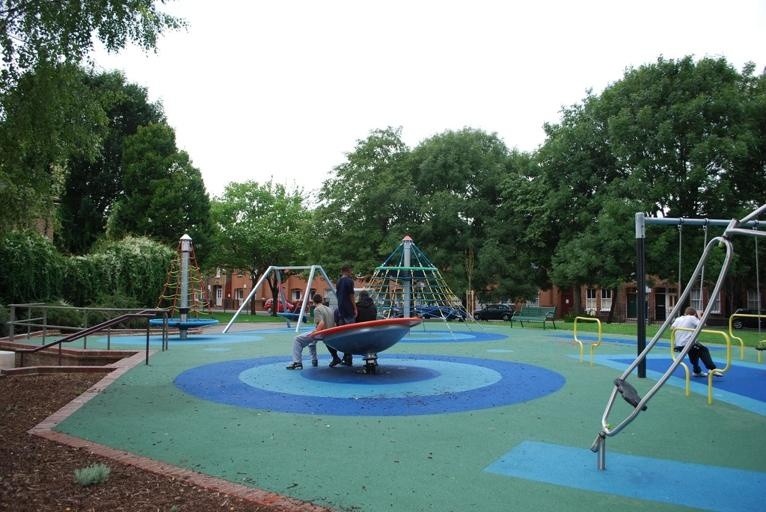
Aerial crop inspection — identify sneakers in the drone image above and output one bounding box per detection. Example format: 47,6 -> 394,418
328,357 -> 341,367
708,369 -> 722,377
312,360 -> 318,368
286,362 -> 302,370
692,371 -> 708,377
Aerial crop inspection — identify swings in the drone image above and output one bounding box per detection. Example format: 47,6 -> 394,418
753,220 -> 766,350
672,218 -> 709,352
273,266 -> 308,319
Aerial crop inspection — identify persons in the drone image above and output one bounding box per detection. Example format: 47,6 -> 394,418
286,265 -> 378,370
670,307 -> 726,378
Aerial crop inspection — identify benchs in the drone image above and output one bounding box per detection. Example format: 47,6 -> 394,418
510,306 -> 557,330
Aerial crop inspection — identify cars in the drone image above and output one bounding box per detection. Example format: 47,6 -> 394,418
264,298 -> 295,313
474,303 -> 514,321
732,308 -> 766,329
